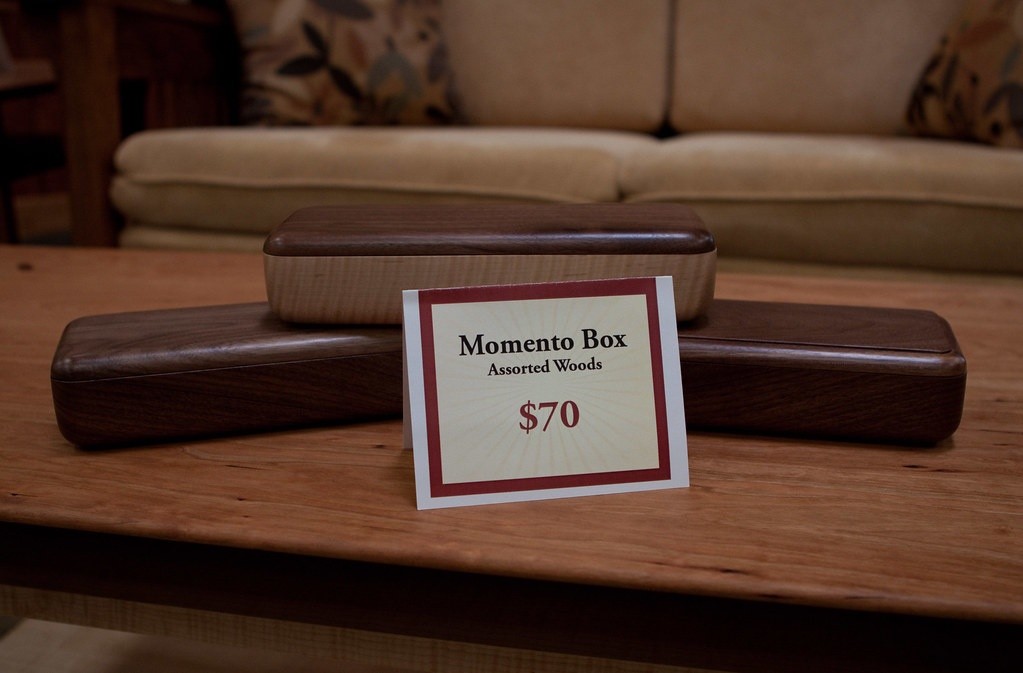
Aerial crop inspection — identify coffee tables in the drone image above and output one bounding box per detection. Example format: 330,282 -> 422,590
1,247 -> 1023,673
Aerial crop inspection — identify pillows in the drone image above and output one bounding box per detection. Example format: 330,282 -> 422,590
896,0 -> 1023,150
230,0 -> 466,127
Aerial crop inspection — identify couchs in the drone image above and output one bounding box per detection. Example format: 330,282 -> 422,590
108,0 -> 1023,274
